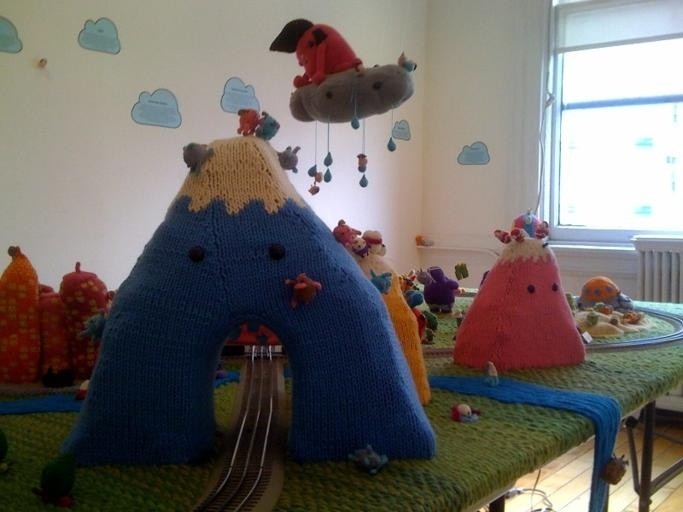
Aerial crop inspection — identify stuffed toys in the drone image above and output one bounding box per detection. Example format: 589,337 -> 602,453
1,19 -> 649,461
1,18 -> 648,462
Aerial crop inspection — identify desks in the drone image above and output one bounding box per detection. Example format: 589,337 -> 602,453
1,279 -> 682,512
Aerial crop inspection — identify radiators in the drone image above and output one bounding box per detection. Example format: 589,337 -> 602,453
631,232 -> 683,399
